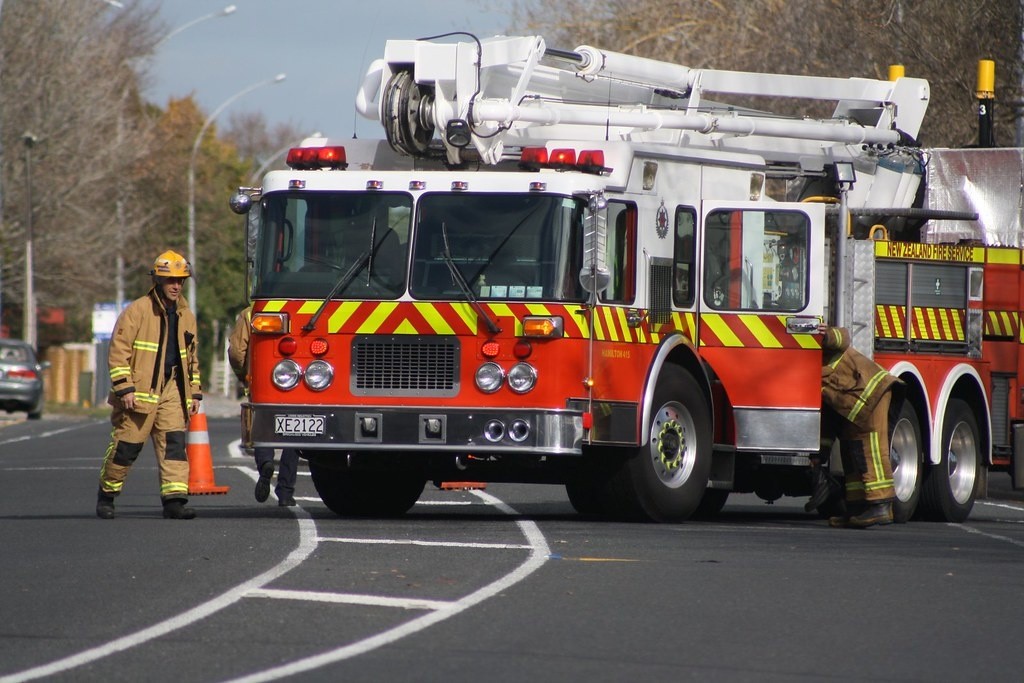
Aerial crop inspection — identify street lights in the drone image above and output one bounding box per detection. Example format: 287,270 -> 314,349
110,5 -> 242,323
181,70 -> 324,321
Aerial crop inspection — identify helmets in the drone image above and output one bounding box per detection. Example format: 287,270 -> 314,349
151,250 -> 191,278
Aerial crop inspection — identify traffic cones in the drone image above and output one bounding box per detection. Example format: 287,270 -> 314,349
188,399 -> 225,495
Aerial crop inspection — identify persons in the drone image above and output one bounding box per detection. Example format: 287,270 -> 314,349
226,275 -> 300,507
96,250 -> 202,518
809,321 -> 898,528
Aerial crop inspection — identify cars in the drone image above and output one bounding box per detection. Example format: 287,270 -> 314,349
0,339 -> 53,418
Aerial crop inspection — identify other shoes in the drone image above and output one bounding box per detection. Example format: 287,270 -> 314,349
255,462 -> 274,502
849,501 -> 894,527
96,489 -> 115,519
163,499 -> 196,519
279,497 -> 296,506
829,503 -> 864,528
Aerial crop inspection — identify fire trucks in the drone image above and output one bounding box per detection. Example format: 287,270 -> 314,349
228,28 -> 1024,529
